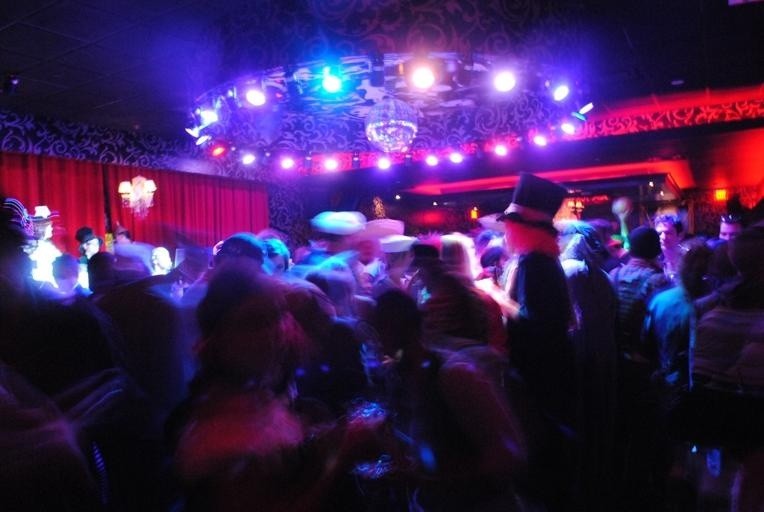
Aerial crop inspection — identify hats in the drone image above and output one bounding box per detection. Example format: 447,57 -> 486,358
496,171 -> 568,238
76,227 -> 103,248
355,219 -> 405,241
379,235 -> 418,252
28,204 -> 61,221
0,196 -> 46,239
310,210 -> 367,235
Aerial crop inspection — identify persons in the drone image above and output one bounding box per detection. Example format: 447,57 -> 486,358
1,170 -> 763,511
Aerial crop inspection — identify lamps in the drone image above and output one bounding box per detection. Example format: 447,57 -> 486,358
117,157 -> 157,221
239,148 -> 255,165
184,120 -> 206,137
363,92 -> 419,153
207,139 -> 226,159
543,77 -> 569,101
574,95 -> 594,115
192,132 -> 209,145
